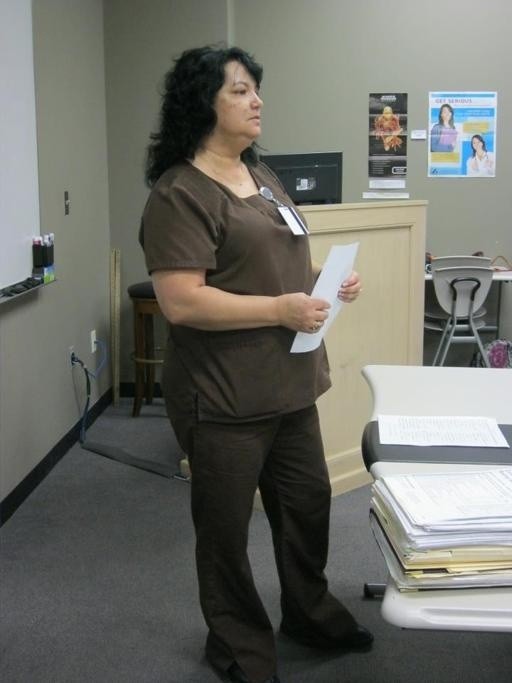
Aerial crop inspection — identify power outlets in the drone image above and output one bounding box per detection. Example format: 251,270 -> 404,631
91,330 -> 98,352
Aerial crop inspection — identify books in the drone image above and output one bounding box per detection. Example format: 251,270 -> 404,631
368,466 -> 512,594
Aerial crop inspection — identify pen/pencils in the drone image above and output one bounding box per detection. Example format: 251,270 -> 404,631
32,232 -> 55,279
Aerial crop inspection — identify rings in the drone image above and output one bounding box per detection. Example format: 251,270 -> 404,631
313,322 -> 320,332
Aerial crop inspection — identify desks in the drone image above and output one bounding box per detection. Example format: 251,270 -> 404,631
180,199 -> 433,510
426,255 -> 512,368
359,364 -> 512,642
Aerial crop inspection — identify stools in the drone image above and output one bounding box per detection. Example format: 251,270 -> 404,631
127,280 -> 168,418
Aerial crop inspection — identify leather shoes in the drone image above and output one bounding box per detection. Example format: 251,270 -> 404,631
205,653 -> 278,682
280,614 -> 373,653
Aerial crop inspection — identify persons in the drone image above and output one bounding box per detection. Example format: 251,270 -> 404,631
137,42 -> 375,682
431,104 -> 494,176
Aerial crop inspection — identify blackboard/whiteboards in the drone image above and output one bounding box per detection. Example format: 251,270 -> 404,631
0,0 -> 54,303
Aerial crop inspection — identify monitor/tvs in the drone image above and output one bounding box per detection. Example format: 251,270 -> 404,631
259,152 -> 343,206
